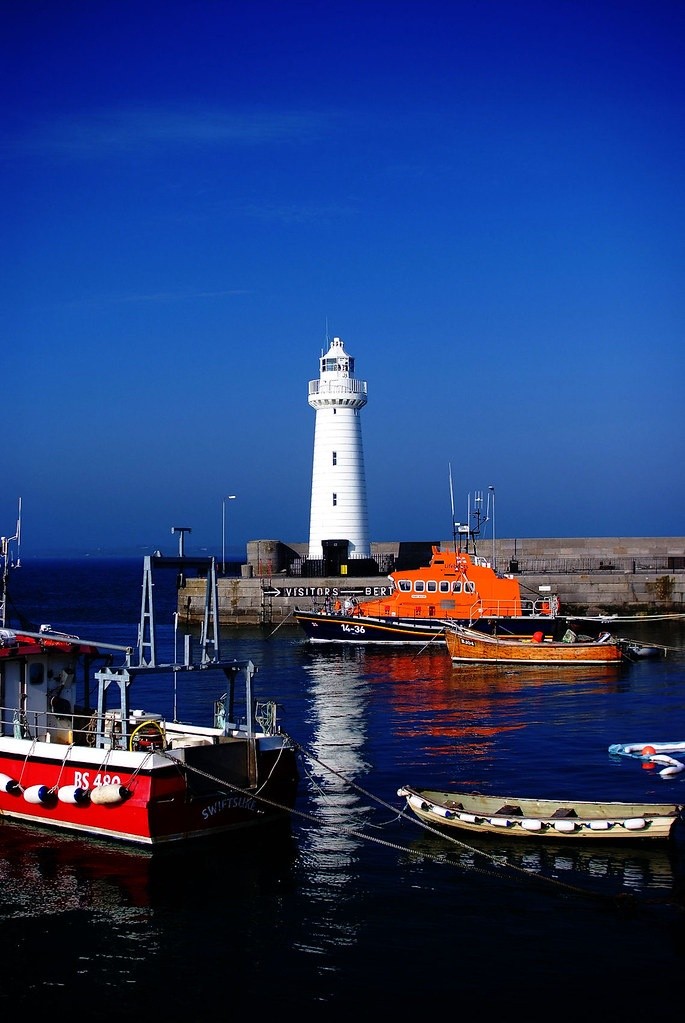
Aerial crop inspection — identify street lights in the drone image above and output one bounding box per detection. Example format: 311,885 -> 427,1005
488,485 -> 497,571
222,496 -> 237,574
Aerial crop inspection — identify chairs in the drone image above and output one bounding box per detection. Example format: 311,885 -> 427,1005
596,632 -> 611,642
531,631 -> 544,643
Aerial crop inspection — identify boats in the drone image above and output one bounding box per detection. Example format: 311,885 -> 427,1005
439,618 -> 661,666
0,498 -> 300,851
396,784 -> 685,842
291,462 -> 685,652
609,740 -> 685,776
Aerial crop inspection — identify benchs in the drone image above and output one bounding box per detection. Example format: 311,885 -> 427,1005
494,804 -> 519,815
444,800 -> 460,808
550,808 -> 574,817
